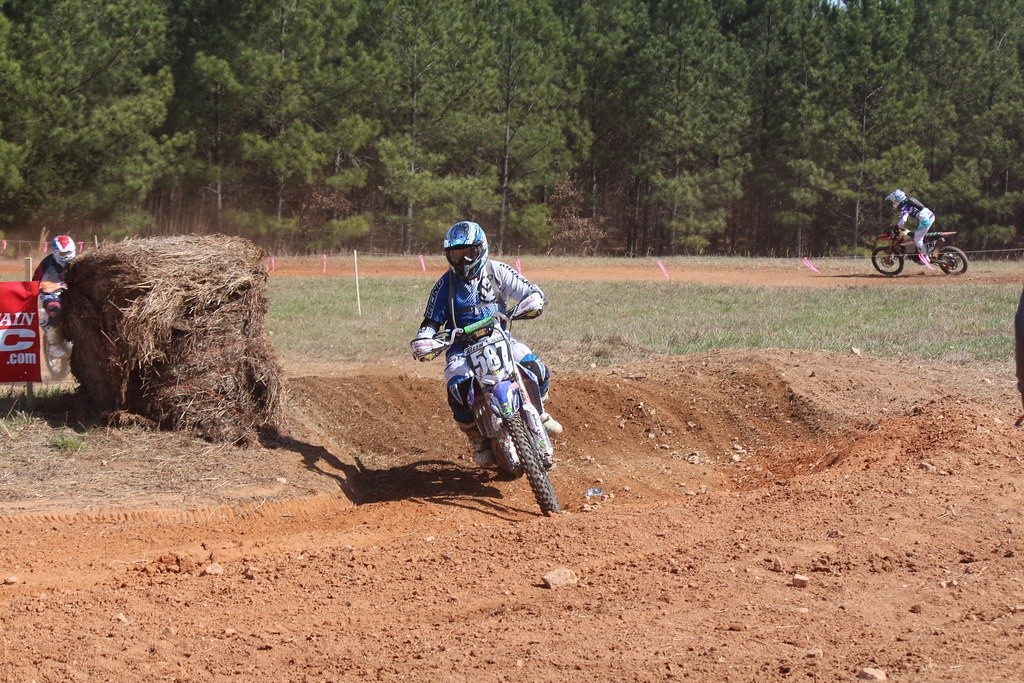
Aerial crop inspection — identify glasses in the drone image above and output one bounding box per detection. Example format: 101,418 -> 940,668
445,242 -> 483,267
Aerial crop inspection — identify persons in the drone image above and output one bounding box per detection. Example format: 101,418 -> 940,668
412,221 -> 562,469
1014,287 -> 1024,425
32,235 -> 76,329
886,189 -> 935,264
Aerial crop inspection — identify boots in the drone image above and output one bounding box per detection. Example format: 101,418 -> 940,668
538,387 -> 563,435
456,420 -> 495,469
916,245 -> 930,264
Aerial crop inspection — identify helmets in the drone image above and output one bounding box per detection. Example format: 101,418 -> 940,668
884,188 -> 909,211
52,234 -> 75,269
443,220 -> 489,281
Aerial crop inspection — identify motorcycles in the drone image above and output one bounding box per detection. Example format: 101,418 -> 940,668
870,215 -> 969,275
413,298 -> 561,517
40,282 -> 72,380
